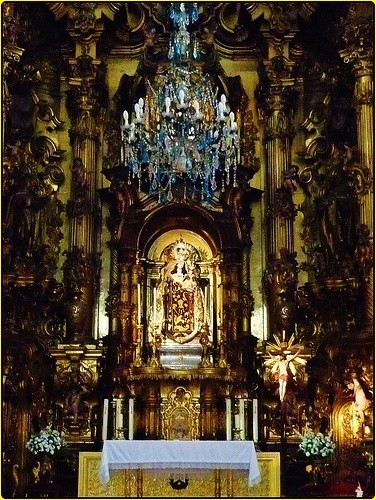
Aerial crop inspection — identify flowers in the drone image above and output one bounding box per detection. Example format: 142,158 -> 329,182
296,429 -> 335,457
25,429 -> 63,454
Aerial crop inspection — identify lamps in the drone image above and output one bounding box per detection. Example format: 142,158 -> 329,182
118,2 -> 241,204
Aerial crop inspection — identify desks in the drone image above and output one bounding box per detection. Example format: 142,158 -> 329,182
100,440 -> 261,497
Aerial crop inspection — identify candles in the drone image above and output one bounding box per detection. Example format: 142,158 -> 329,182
239,399 -> 244,440
252,399 -> 258,443
103,399 -> 109,441
226,399 -> 232,440
116,400 -> 123,428
128,399 -> 134,440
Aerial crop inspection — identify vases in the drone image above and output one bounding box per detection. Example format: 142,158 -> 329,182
29,452 -> 63,498
301,459 -> 332,498
